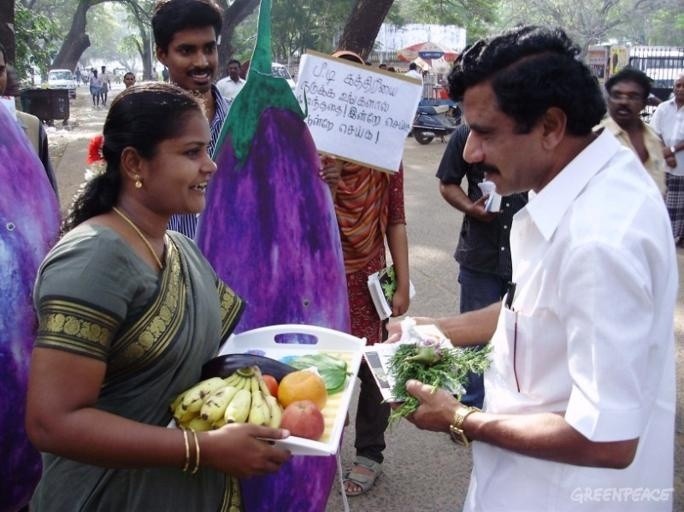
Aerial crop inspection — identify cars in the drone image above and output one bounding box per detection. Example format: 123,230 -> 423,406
81,65 -> 144,81
270,62 -> 296,99
17,66 -> 41,89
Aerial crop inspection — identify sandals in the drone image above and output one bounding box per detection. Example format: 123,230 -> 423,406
340,456 -> 382,496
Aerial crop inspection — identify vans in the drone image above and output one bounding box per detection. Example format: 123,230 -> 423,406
45,69 -> 76,100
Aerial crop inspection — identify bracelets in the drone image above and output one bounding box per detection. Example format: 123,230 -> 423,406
187,427 -> 200,475
182,430 -> 190,473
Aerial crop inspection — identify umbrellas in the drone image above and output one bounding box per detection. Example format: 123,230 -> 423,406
396,41 -> 459,73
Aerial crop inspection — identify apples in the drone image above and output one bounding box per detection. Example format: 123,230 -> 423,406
278,400 -> 325,440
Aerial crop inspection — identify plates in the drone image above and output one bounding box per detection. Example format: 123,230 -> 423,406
163,323 -> 367,459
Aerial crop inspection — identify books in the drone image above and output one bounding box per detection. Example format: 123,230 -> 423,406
367,265 -> 416,321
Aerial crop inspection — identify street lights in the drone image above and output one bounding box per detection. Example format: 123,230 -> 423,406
147,27 -> 154,82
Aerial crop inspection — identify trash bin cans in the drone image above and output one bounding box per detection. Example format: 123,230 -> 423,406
21,88 -> 70,126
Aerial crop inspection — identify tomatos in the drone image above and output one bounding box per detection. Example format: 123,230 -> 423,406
263,375 -> 278,397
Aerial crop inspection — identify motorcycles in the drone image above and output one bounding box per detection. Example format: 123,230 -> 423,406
410,104 -> 465,145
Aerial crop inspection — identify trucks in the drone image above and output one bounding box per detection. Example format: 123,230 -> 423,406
629,45 -> 683,116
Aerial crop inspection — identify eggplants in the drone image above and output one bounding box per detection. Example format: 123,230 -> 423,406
202,353 -> 299,382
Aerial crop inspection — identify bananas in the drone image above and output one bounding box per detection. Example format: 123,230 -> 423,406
168,366 -> 282,431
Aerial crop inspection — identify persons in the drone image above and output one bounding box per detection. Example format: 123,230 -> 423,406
0,45 -> 60,206
26,80 -> 293,512
152,0 -> 340,241
436,125 -> 529,408
598,68 -> 667,198
75,60 -> 449,137
649,76 -> 684,247
383,27 -> 679,512
291,50 -> 410,497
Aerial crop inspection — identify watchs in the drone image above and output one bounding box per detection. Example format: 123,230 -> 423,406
449,407 -> 481,447
671,144 -> 675,154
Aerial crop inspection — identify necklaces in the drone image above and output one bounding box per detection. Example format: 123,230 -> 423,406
112,207 -> 163,270
